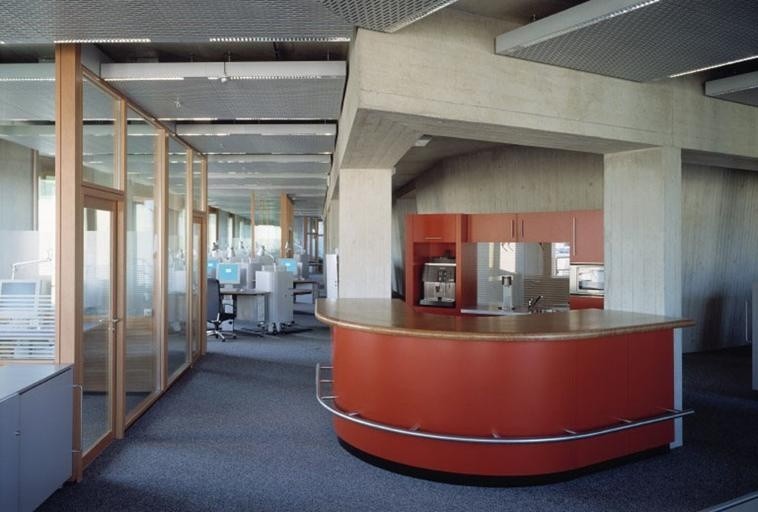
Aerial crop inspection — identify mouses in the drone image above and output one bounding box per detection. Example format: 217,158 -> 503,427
28,326 -> 40,330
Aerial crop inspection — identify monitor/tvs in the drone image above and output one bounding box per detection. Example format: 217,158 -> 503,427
0,279 -> 41,328
276,257 -> 298,276
216,262 -> 240,292
204,259 -> 220,273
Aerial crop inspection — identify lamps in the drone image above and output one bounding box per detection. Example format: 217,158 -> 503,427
210,240 -> 304,261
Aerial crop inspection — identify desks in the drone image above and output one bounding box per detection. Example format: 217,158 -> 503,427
223,278 -> 318,335
1,322 -> 99,355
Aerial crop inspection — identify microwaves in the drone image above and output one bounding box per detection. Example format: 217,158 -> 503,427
568,265 -> 605,296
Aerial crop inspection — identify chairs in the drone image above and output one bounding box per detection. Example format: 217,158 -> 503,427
207,278 -> 235,342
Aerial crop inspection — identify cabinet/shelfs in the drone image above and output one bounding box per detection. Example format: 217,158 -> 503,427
571,210 -> 604,265
413,215 -> 458,312
467,212 -> 570,242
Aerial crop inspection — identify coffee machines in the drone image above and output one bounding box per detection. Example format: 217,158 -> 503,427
419,249 -> 456,307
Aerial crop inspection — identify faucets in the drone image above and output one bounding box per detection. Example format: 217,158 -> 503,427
527,295 -> 544,314
